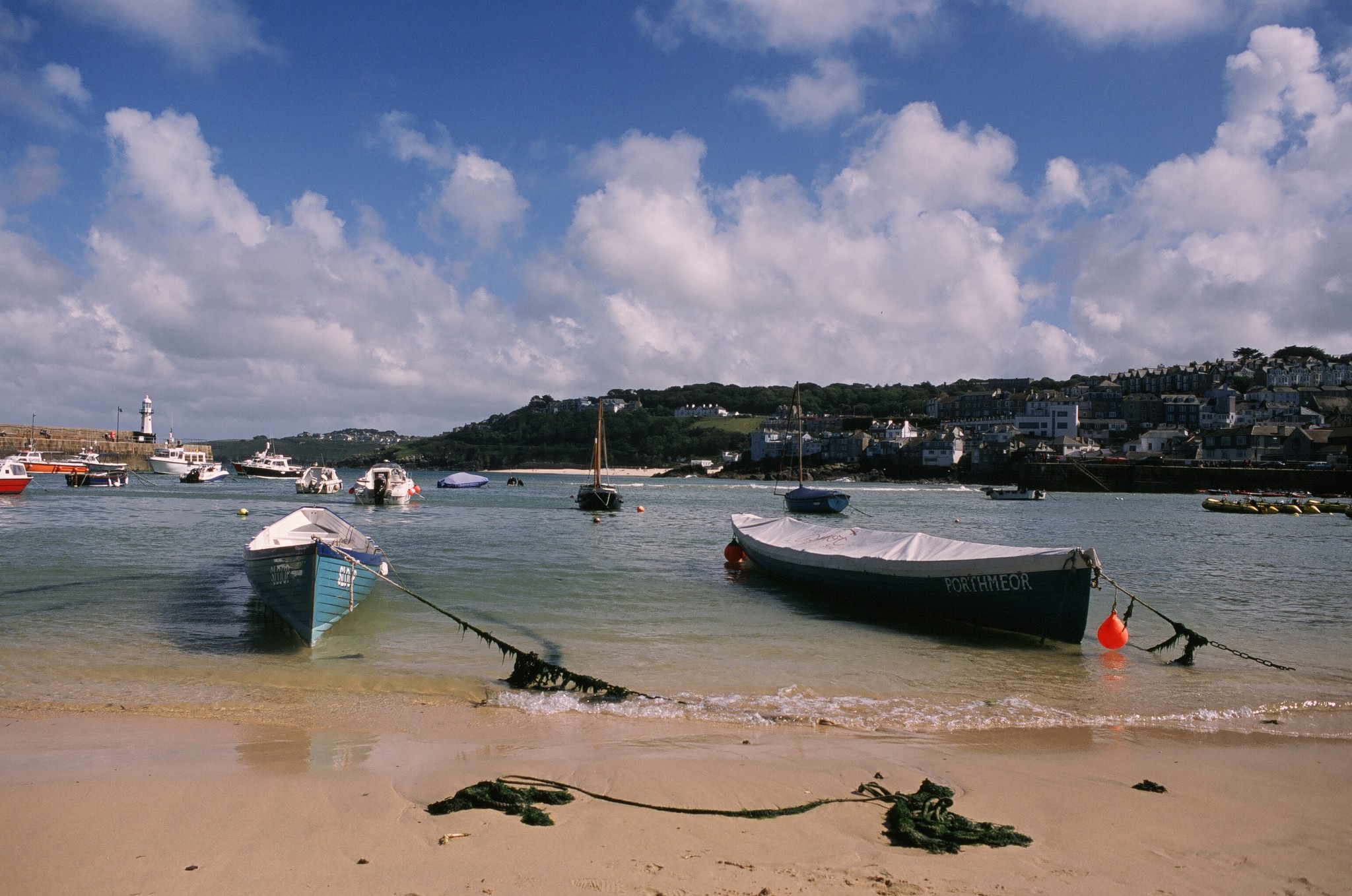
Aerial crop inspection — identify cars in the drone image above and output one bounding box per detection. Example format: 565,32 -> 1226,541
1259,461 -> 1286,469
0,459 -> 34,494
1305,462 -> 1332,471
2,437 -> 129,488
1134,456 -> 1164,467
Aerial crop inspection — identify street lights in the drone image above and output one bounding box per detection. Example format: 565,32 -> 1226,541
117,406 -> 123,442
32,414 -> 36,438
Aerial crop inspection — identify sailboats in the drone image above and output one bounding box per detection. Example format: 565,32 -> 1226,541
574,399 -> 624,513
773,382 -> 851,515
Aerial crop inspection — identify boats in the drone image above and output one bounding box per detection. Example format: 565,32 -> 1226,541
1202,492 -> 1351,517
1197,488 -> 1345,498
437,472 -> 490,489
241,505 -> 389,649
730,512 -> 1100,645
295,453 -> 343,494
987,486 -> 1046,500
349,459 -> 421,505
146,411 -> 229,484
228,430 -> 306,479
842,478 -> 855,482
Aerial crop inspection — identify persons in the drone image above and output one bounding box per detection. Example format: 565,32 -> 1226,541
507,476 -> 524,486
1024,451 -> 1051,463
105,433 -> 113,442
40,429 -> 50,439
1336,500 -> 1340,504
1280,499 -> 1284,504
1196,457 -> 1254,469
